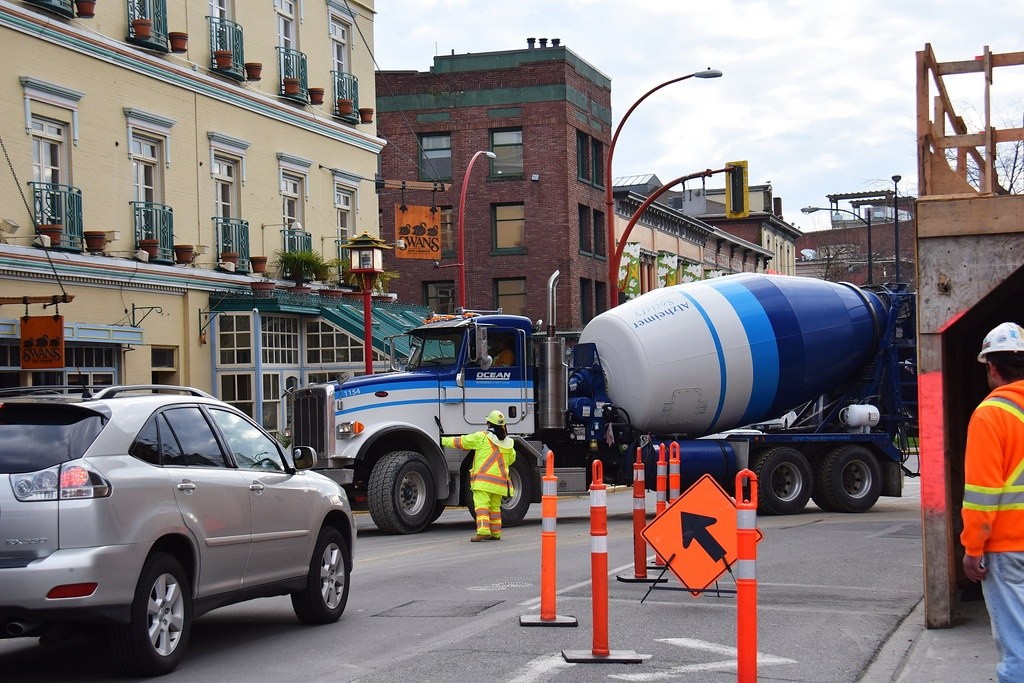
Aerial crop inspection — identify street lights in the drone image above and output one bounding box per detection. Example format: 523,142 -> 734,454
800,205 -> 874,287
892,174 -> 901,284
460,149 -> 497,314
607,69 -> 733,299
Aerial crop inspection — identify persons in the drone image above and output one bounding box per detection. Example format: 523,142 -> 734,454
441,410 -> 516,542
960,321 -> 1024,683
491,338 -> 515,366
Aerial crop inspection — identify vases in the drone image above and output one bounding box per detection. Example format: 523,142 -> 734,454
71,0 -> 375,125
247,282 -> 393,302
35,224 -> 268,274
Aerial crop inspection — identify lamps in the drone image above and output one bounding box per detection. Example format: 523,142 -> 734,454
1,235 -> 52,249
384,239 -> 405,249
321,234 -> 357,241
262,221 -> 303,231
104,248 -> 150,263
0,218 -> 20,233
195,260 -> 235,273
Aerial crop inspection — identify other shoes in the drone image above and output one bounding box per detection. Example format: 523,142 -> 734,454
489,536 -> 500,540
471,534 -> 491,542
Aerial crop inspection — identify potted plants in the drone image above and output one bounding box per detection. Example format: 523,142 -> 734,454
272,247 -> 336,294
335,253 -> 399,299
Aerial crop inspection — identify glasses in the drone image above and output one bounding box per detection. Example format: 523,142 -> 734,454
494,338 -> 502,342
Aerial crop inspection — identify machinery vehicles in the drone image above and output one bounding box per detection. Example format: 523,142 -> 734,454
324,270 -> 907,533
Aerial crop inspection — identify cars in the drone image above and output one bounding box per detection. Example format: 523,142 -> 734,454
0,381 -> 354,665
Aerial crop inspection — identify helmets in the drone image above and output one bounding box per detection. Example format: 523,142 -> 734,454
485,410 -> 506,426
977,322 -> 1024,364
